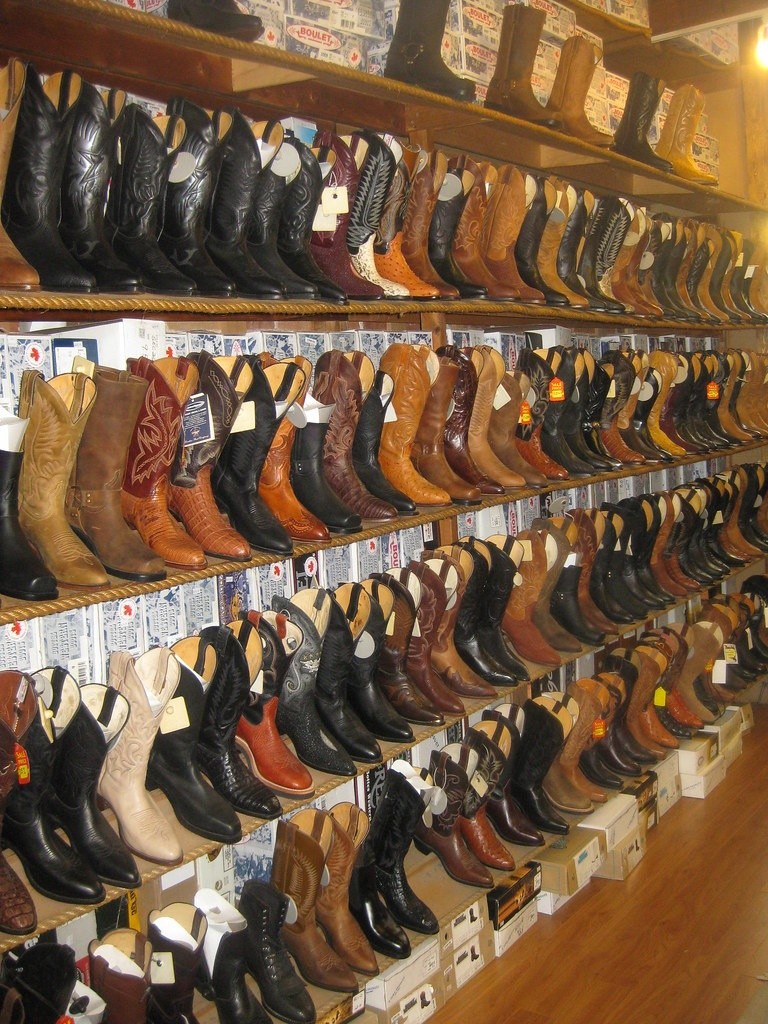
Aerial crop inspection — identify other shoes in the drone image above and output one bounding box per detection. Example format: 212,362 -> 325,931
168,0 -> 265,41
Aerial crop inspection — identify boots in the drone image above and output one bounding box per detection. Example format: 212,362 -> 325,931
545,35 -> 616,146
0,68 -> 768,1024
484,4 -> 563,125
383,0 -> 477,102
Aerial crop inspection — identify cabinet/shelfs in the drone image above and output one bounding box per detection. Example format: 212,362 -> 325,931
0,2 -> 768,1024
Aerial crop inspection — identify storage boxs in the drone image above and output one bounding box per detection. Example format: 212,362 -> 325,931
327,699 -> 755,1023
